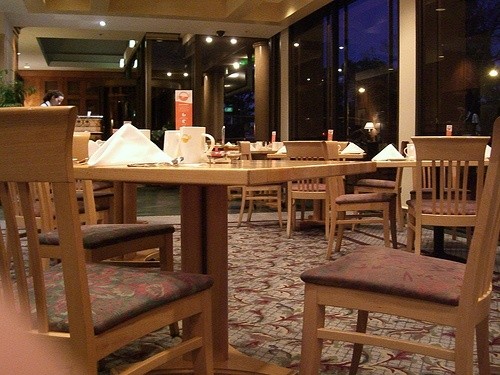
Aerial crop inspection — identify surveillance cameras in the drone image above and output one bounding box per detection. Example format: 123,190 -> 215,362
216,31 -> 225,38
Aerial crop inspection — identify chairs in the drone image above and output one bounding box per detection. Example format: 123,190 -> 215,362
0,106 -> 215,375
300,115 -> 500,375
0,132 -> 490,337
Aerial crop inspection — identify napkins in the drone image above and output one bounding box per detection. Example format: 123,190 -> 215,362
485,145 -> 492,159
88,141 -> 100,158
277,146 -> 287,154
225,142 -> 232,146
87,123 -> 174,167
372,143 -> 404,160
341,143 -> 365,154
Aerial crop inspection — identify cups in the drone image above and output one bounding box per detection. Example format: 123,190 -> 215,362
163,131 -> 181,158
179,126 -> 215,167
403,144 -> 416,161
272,142 -> 283,151
256,142 -> 263,151
140,129 -> 150,140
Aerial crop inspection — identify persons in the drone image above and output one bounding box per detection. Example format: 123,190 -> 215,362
39,90 -> 64,107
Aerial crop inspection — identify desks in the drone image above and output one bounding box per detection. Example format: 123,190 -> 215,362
267,154 -> 364,229
70,159 -> 377,375
372,159 -> 491,189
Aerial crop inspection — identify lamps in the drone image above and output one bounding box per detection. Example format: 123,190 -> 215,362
364,122 -> 374,132
